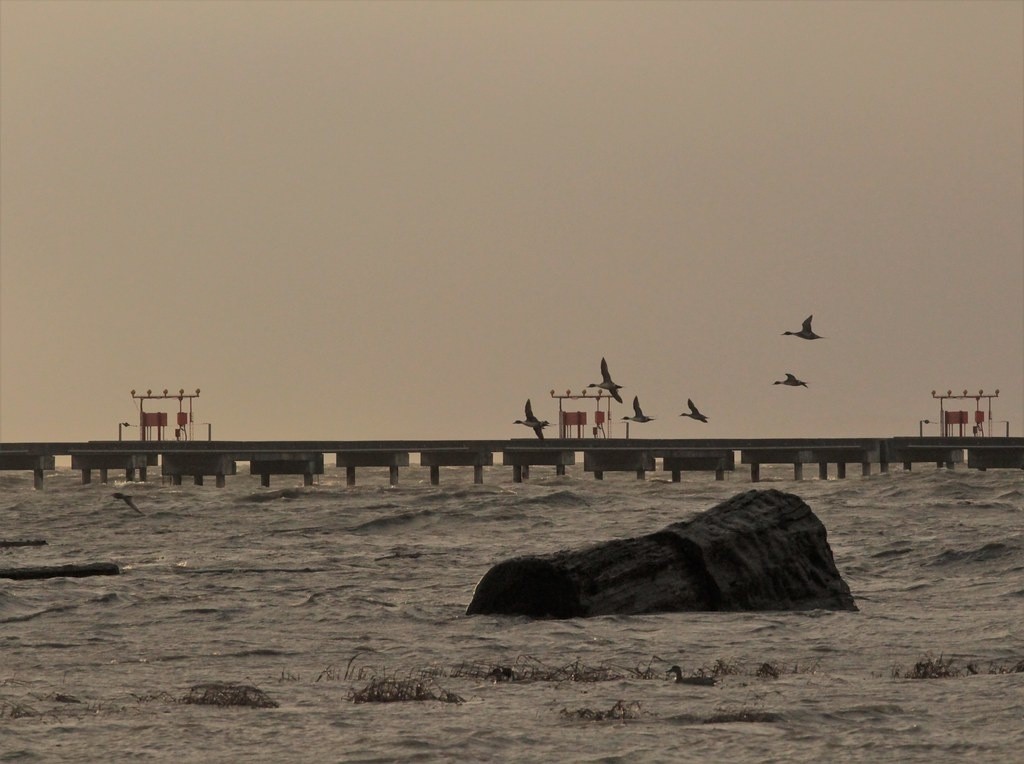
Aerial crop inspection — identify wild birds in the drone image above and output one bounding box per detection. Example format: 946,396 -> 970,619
511,398 -> 550,440
585,356 -> 624,405
772,373 -> 809,389
104,492 -> 142,514
620,395 -> 656,423
679,398 -> 710,424
780,314 -> 825,340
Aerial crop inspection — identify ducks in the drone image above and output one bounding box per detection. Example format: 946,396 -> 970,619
664,665 -> 718,685
